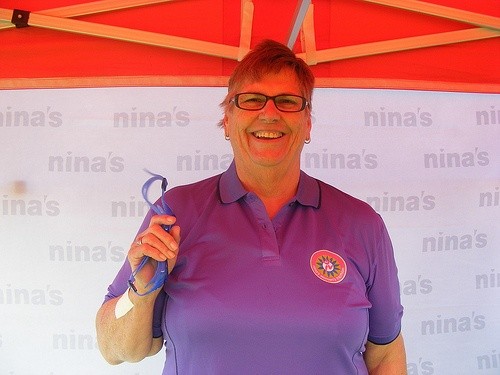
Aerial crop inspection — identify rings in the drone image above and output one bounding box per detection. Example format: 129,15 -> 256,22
136,238 -> 142,246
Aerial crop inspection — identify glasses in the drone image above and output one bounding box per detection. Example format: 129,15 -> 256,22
127,168 -> 176,296
228,92 -> 309,112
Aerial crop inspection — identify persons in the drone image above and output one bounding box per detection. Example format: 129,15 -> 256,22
97,38 -> 407,375
